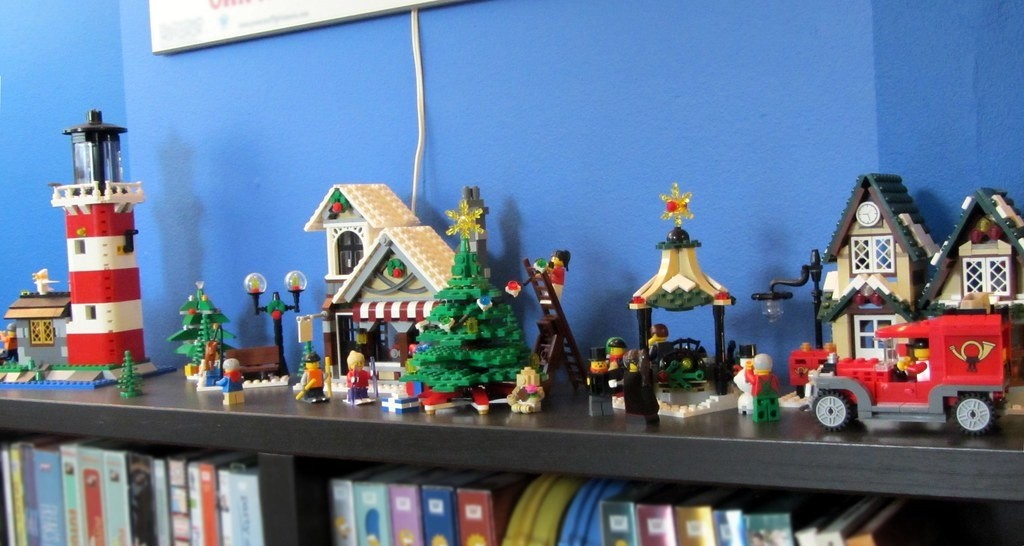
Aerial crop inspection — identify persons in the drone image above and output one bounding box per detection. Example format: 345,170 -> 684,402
536,249 -> 571,304
647,324 -> 668,347
216,358 -> 244,405
745,354 -> 782,422
0,322 -> 18,361
301,352 -> 329,399
898,338 -> 930,381
608,338 -> 627,361
345,351 -> 373,401
589,347 -> 608,373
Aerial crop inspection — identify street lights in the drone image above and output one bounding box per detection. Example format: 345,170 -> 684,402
243,270 -> 307,378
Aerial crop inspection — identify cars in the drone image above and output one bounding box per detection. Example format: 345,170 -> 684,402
807,311 -> 1014,438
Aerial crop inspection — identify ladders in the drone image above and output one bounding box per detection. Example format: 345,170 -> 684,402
522,257 -> 590,391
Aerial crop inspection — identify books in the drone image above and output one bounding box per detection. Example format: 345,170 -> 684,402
0,433 -> 265,546
600,479 -> 907,546
330,463 -> 536,546
